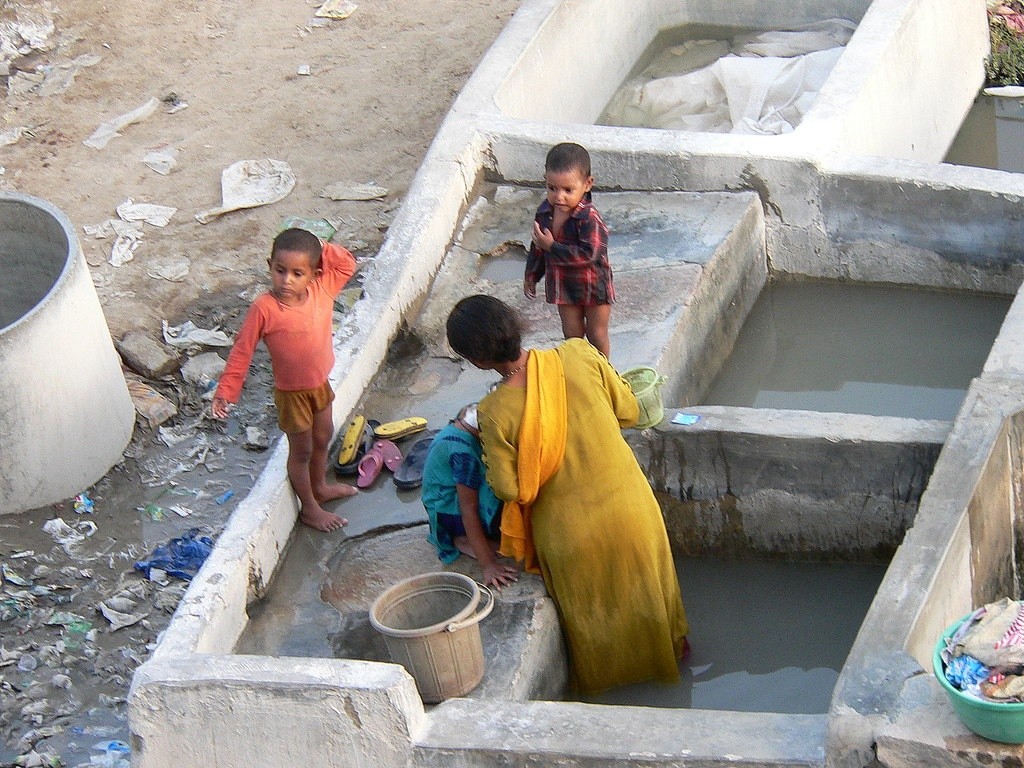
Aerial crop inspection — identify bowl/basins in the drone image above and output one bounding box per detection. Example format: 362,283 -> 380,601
932,600 -> 1024,745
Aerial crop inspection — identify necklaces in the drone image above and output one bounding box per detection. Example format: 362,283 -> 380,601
503,364 -> 527,379
457,418 -> 475,436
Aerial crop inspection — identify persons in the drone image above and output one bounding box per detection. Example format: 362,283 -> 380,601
210,226 -> 360,533
419,403 -> 519,592
522,141 -> 617,360
446,294 -> 689,694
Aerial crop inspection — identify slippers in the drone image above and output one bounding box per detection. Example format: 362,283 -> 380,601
338,415 -> 369,465
393,428 -> 443,489
364,419 -> 382,436
375,417 -> 427,440
373,439 -> 404,472
357,450 -> 383,489
334,438 -> 373,472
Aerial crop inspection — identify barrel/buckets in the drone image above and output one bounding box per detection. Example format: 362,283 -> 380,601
619,366 -> 670,430
368,572 -> 495,704
